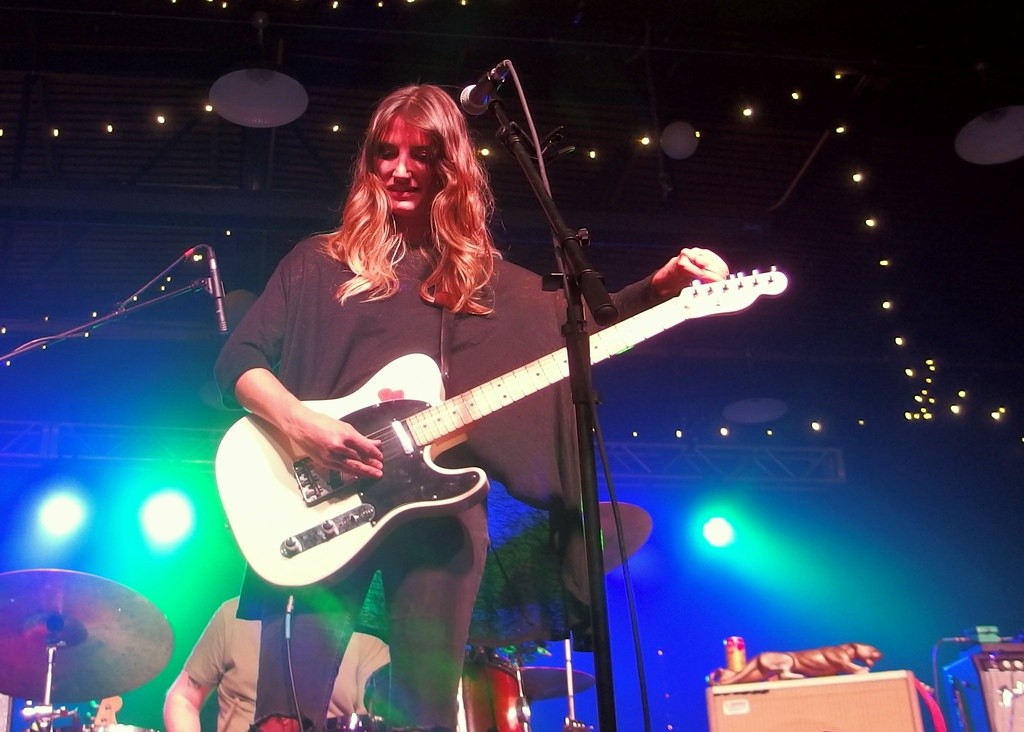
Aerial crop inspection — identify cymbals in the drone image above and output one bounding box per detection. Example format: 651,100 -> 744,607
476,501 -> 655,607
520,659 -> 596,704
2,565 -> 177,705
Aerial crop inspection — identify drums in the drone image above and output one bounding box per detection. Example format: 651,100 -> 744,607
361,651 -> 532,732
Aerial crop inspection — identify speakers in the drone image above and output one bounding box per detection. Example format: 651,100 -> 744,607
941,652 -> 1024,732
704,670 -> 926,732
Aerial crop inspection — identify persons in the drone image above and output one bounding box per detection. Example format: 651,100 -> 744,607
216,85 -> 729,732
163,593 -> 391,732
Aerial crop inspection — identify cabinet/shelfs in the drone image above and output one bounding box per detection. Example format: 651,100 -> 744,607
706,670 -> 926,732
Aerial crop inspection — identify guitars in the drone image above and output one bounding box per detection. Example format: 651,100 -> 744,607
212,262 -> 791,597
82,695 -> 123,732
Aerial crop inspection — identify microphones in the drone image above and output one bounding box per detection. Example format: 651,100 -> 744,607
206,246 -> 228,332
460,60 -> 512,115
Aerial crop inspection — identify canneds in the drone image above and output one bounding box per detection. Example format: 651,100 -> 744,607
724,636 -> 747,672
